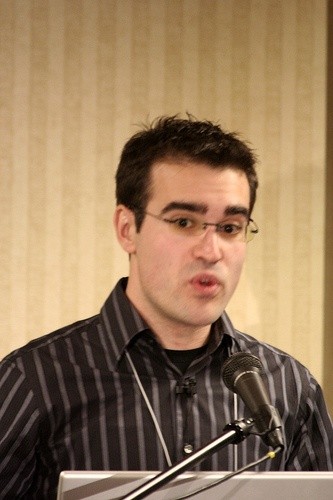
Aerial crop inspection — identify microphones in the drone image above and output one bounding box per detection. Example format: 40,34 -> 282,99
221,352 -> 285,455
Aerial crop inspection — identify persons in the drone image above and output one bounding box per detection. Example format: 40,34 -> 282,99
1,113 -> 333,500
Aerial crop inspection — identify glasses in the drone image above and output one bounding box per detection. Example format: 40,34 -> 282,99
129,205 -> 259,240
